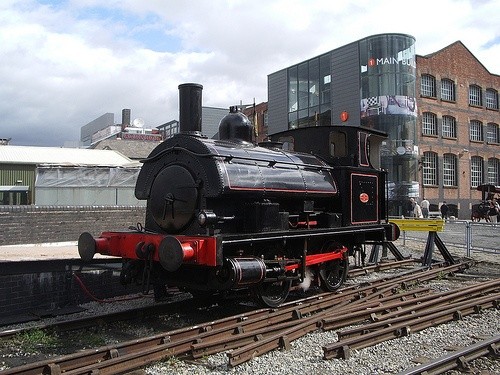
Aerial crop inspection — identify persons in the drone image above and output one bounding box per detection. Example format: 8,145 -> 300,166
440,201 -> 449,223
408,202 -> 414,217
420,197 -> 431,219
413,202 -> 424,219
486,199 -> 500,228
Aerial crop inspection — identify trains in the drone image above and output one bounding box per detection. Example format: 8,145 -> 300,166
76,83 -> 401,314
